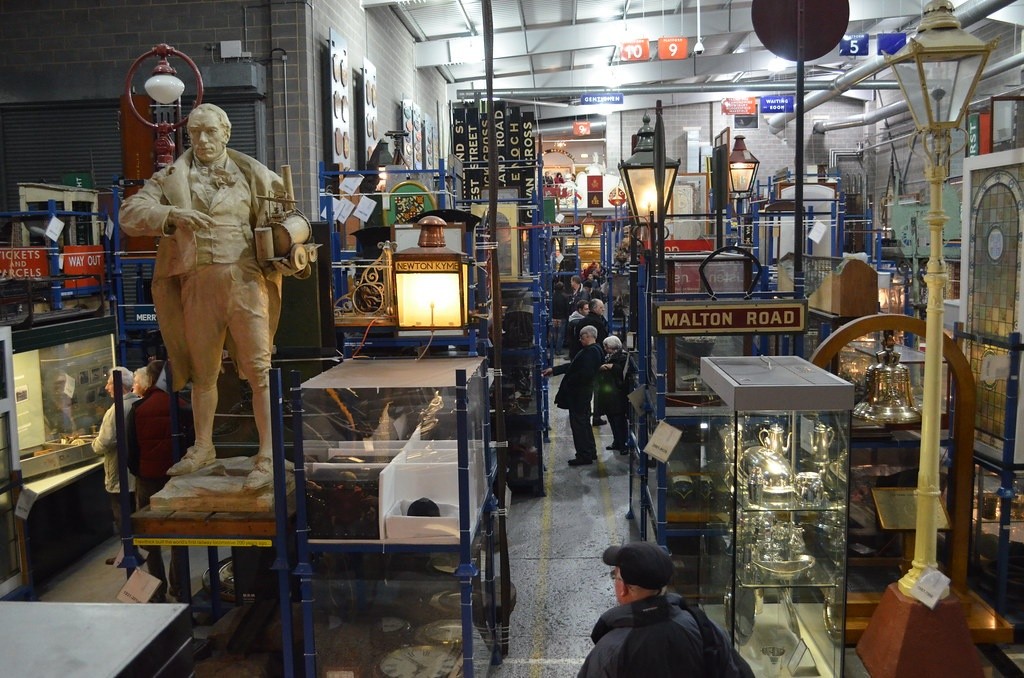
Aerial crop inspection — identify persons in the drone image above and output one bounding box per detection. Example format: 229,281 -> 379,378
599,335 -> 639,455
553,259 -> 609,426
542,171 -> 553,187
541,325 -> 605,466
92,360 -> 195,603
553,172 -> 565,184
118,103 -> 285,490
577,542 -> 755,678
571,173 -> 576,182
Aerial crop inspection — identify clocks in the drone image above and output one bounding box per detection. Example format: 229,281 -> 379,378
321,587 -> 479,677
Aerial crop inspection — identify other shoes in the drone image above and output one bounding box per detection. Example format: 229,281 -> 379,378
556,352 -> 565,355
569,458 -> 593,465
620,448 -> 629,455
564,357 -> 570,360
106,556 -> 116,565
593,455 -> 597,460
593,419 -> 607,426
606,444 -> 617,449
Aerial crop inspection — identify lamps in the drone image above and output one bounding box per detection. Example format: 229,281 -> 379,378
729,136 -> 760,199
582,210 -> 598,240
391,215 -> 470,338
617,112 -> 682,227
126,45 -> 204,135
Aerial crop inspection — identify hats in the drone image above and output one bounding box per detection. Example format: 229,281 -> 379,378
604,542 -> 673,590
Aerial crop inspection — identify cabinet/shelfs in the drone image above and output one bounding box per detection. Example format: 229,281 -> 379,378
113,360 -> 305,678
602,174 -> 1024,678
290,342 -> 514,678
319,161 -> 581,496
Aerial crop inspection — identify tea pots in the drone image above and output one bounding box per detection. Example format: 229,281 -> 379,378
809,422 -> 834,460
759,423 -> 792,457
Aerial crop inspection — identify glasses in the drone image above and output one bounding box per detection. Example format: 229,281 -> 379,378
610,570 -> 625,582
579,333 -> 584,337
